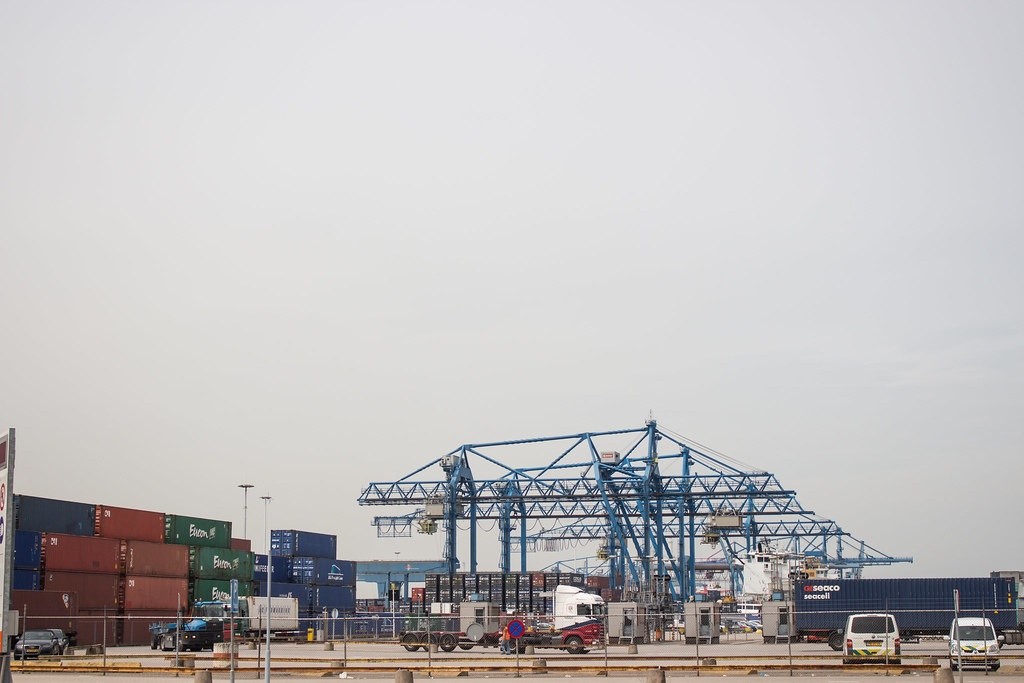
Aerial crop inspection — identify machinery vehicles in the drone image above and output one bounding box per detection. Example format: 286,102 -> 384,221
146,617 -> 223,652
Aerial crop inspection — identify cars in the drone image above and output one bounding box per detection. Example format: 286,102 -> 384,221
49,629 -> 70,655
12,629 -> 61,661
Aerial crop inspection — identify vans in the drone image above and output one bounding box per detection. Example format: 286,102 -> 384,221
942,616 -> 1005,672
836,611 -> 902,665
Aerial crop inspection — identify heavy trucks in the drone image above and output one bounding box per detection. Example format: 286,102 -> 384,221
396,583 -> 606,655
793,575 -> 1024,651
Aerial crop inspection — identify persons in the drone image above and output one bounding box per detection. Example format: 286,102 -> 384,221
500,623 -> 511,655
62,626 -> 79,648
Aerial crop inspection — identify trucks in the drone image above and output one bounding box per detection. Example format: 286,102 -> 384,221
188,592 -> 299,642
